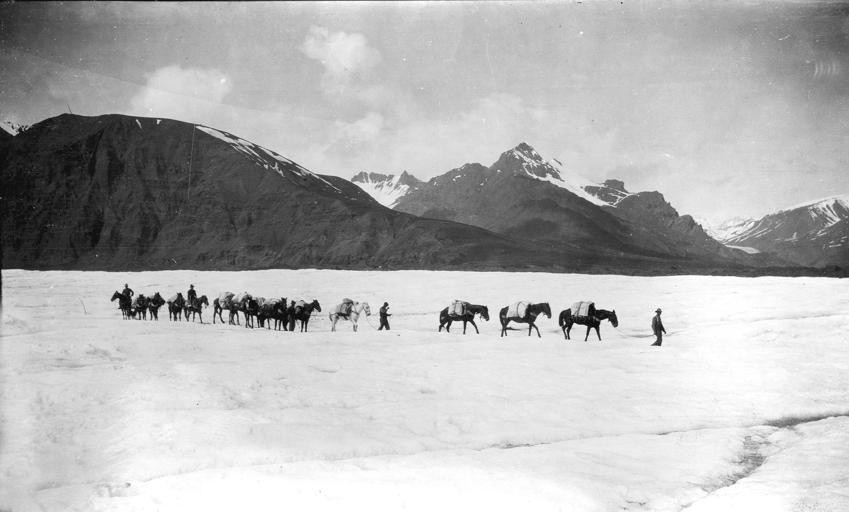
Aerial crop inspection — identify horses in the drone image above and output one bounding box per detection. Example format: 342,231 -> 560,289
327,300 -> 372,331
439,303 -> 490,334
212,295 -> 323,333
498,301 -> 553,337
558,307 -> 619,340
108,291 -> 209,323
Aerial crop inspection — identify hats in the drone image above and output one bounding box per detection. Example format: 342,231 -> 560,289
190,284 -> 195,288
655,308 -> 662,313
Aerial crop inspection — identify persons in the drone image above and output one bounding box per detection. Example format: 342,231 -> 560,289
377,302 -> 391,329
187,283 -> 197,303
650,308 -> 667,346
285,301 -> 296,331
118,284 -> 134,309
